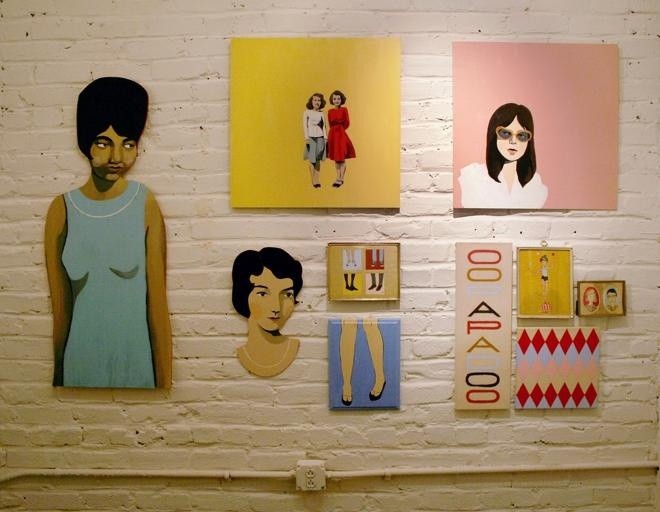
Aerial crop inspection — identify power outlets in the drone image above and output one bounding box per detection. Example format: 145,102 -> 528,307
294,459 -> 328,494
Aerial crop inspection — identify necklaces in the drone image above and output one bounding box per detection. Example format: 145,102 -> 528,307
68,183 -> 140,218
242,339 -> 292,368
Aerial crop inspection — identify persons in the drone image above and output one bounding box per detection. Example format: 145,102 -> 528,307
585,288 -> 599,311
606,288 -> 619,311
339,317 -> 386,403
456,103 -> 550,208
46,78 -> 174,389
342,246 -> 384,293
304,93 -> 328,189
225,247 -> 304,377
538,255 -> 551,294
326,90 -> 356,188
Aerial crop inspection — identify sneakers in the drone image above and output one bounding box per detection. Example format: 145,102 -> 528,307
346,261 -> 358,267
370,260 -> 384,268
345,285 -> 359,291
368,284 -> 376,289
375,283 -> 383,292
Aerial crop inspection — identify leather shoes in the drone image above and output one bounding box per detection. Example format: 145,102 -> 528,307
313,180 -> 321,189
341,385 -> 353,406
332,179 -> 343,188
368,380 -> 386,401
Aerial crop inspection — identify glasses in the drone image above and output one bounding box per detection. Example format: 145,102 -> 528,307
496,126 -> 534,143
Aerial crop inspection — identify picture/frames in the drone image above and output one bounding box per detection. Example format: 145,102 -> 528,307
516,245 -> 575,320
576,279 -> 626,317
328,241 -> 402,303
